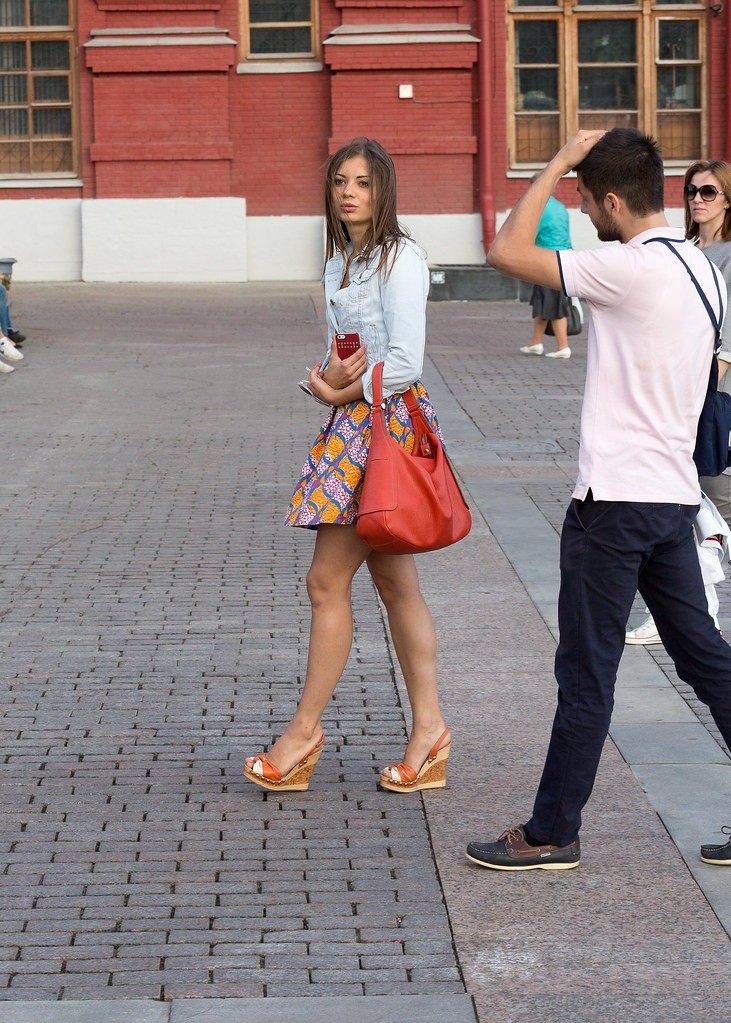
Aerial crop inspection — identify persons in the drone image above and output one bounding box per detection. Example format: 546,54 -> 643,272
240,137 -> 449,792
519,172 -> 571,358
467,126 -> 731,871
0,284 -> 26,374
624,160 -> 731,646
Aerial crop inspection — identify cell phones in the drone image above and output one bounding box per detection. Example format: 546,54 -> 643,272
335,333 -> 360,361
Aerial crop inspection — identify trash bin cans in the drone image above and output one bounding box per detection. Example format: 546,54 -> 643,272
0,258 -> 18,274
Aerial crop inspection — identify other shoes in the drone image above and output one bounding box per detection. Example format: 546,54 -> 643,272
8,331 -> 26,344
545,348 -> 572,359
14,345 -> 23,348
520,343 -> 544,355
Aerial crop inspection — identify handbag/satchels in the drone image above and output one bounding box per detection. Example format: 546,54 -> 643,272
544,305 -> 582,335
356,362 -> 472,555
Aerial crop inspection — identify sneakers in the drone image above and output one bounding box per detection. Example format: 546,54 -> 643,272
700,826 -> 731,866
0,361 -> 14,373
624,607 -> 662,644
465,824 -> 581,869
0,338 -> 24,361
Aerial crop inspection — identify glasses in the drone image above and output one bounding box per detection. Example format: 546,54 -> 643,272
684,184 -> 725,202
296,380 -> 333,409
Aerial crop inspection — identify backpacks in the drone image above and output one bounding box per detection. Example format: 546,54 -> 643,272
642,238 -> 731,477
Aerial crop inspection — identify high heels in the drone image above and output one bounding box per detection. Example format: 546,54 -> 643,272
379,728 -> 451,793
243,734 -> 325,791
